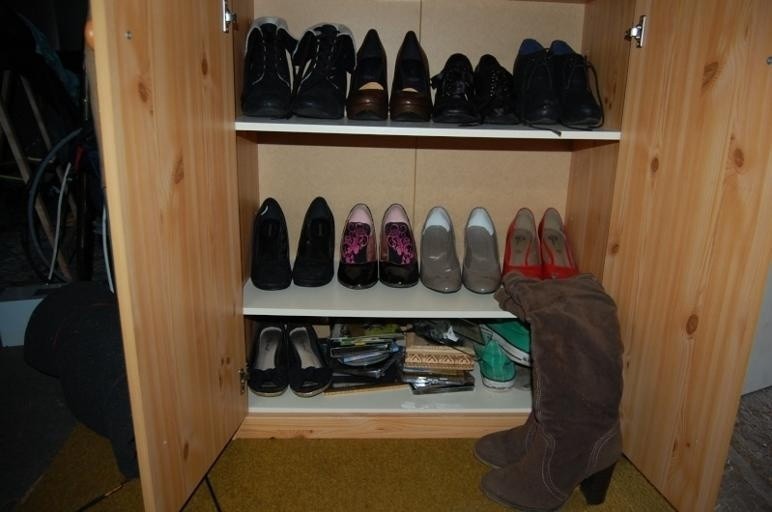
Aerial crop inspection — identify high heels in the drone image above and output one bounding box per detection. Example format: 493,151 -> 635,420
419,207 -> 463,293
514,39 -> 562,137
462,207 -> 502,294
291,197 -> 335,286
503,208 -> 543,281
538,207 -> 580,281
347,29 -> 389,119
549,41 -> 605,131
250,197 -> 292,291
380,203 -> 419,288
390,31 -> 433,121
337,202 -> 379,290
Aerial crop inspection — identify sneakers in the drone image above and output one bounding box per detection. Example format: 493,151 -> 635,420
475,319 -> 532,367
472,339 -> 519,389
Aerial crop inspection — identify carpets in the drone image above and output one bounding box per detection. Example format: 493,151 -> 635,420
12,428 -> 683,512
714,384 -> 772,512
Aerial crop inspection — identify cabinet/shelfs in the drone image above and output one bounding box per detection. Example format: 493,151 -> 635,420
78,1 -> 768,512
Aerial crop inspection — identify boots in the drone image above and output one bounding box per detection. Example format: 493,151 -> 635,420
475,289 -> 534,466
480,271 -> 623,512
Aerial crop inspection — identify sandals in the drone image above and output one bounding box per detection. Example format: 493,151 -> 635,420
247,326 -> 290,397
288,323 -> 333,398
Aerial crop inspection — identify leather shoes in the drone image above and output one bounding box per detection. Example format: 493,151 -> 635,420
431,54 -> 481,124
241,16 -> 297,117
292,22 -> 357,119
475,55 -> 522,125
